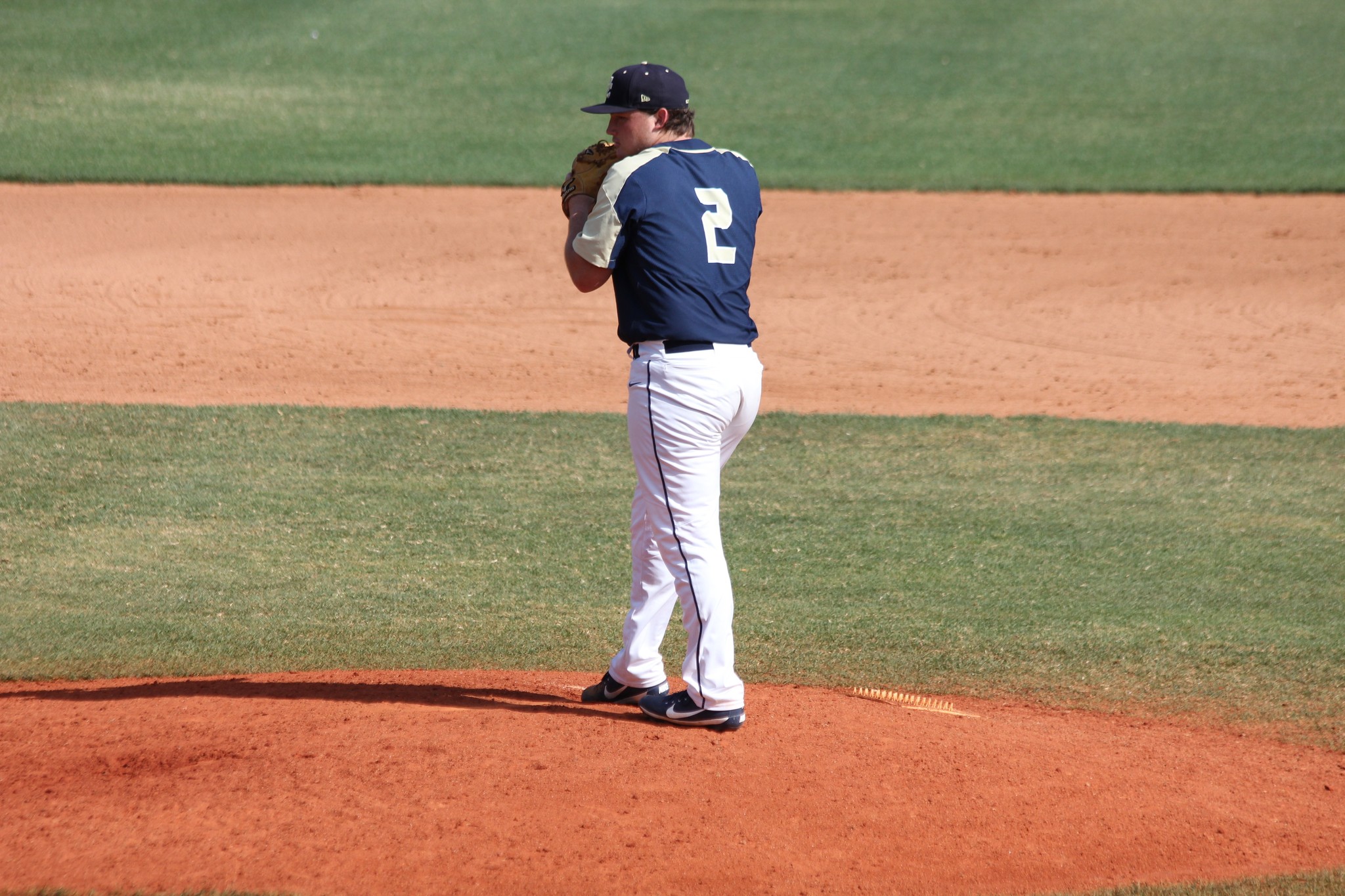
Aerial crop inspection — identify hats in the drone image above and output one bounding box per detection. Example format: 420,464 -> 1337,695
580,61 -> 690,114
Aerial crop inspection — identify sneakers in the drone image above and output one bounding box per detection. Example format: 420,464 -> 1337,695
640,689 -> 746,729
579,672 -> 669,702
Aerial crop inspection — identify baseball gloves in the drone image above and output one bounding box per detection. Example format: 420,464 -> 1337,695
559,140 -> 624,222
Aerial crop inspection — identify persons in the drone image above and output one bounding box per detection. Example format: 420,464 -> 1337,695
561,63 -> 764,730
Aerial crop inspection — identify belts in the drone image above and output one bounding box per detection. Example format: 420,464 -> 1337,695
633,343 -> 752,359
